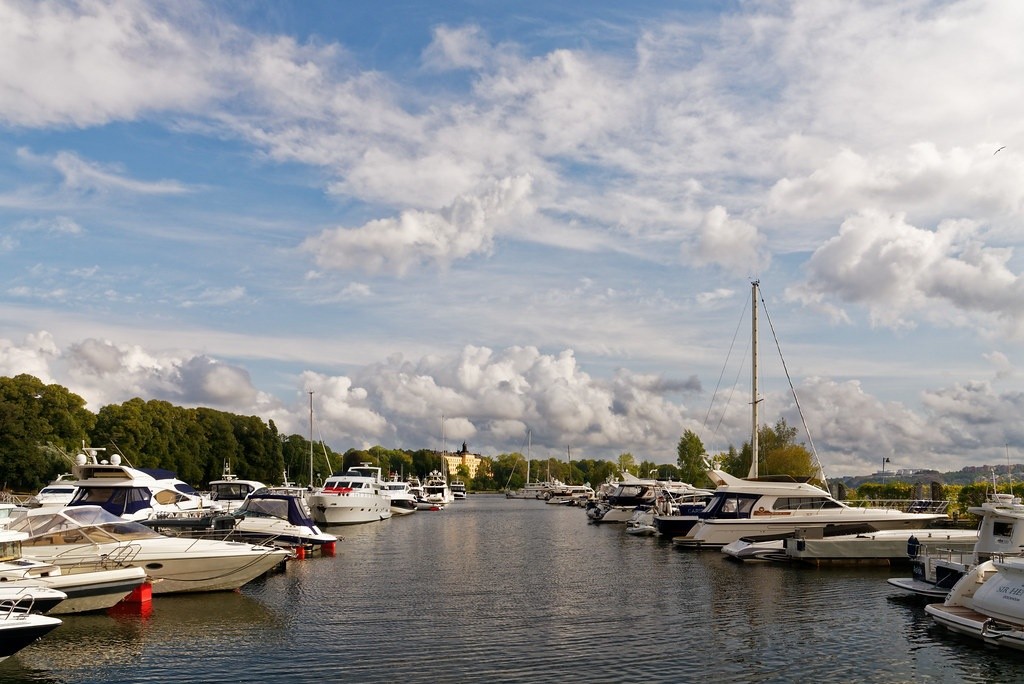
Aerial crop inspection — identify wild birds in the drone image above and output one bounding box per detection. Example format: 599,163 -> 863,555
994,146 -> 1006,155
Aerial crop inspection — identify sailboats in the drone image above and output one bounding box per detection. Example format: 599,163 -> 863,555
440,409 -> 455,501
504,429 -> 541,498
270,388 -> 334,499
652,277 -> 833,535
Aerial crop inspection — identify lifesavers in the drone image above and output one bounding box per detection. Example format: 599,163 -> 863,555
759,507 -> 764,512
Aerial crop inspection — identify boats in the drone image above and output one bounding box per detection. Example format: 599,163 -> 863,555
35,438 -> 392,544
588,454 -> 980,568
885,431 -> 1024,651
0,502 -> 297,677
385,474 -> 595,517
